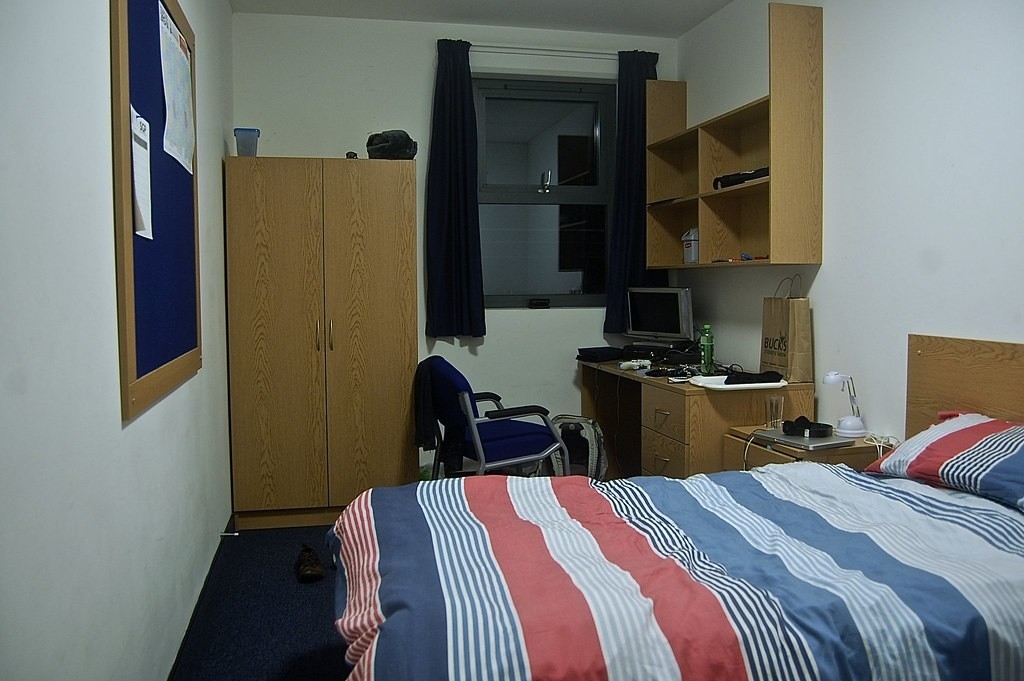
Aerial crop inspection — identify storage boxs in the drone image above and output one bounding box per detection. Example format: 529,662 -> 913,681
234,127 -> 260,157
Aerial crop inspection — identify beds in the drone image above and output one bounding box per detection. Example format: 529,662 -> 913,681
327,334 -> 1024,681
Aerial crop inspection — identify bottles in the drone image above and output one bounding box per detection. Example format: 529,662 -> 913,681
700,325 -> 714,374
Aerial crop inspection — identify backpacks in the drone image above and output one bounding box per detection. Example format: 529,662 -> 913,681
530,413 -> 609,483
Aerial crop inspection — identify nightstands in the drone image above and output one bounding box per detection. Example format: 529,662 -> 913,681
724,423 -> 894,473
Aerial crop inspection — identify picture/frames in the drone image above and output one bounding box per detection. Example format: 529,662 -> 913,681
109,0 -> 203,430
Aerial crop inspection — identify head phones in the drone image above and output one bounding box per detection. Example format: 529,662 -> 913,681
783,416 -> 831,438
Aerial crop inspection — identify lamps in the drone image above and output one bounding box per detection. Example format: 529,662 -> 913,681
822,371 -> 865,438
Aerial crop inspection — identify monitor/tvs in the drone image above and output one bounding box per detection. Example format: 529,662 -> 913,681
627,288 -> 693,348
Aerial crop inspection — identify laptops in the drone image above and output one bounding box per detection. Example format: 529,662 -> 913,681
753,426 -> 855,451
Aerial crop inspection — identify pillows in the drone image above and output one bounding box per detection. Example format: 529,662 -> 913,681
863,410 -> 1024,514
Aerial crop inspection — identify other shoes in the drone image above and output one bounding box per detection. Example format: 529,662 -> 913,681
294,542 -> 322,583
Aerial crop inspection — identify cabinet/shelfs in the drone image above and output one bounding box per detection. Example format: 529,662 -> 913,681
224,154 -> 420,532
576,359 -> 816,480
645,3 -> 822,270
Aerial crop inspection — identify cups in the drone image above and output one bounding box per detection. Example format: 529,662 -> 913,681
765,395 -> 785,429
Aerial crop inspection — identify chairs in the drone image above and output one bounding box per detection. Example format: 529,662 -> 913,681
417,354 -> 571,478
